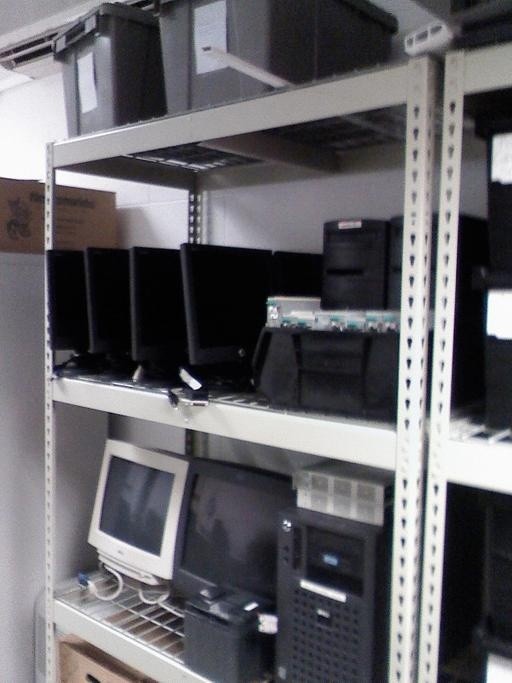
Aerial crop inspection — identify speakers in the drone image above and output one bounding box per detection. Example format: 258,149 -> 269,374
390,209 -> 492,309
319,217 -> 389,312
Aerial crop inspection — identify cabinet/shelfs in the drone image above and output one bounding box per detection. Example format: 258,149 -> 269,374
40,44 -> 477,682
415,44 -> 511,682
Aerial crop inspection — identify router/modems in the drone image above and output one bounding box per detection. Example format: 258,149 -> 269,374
296,458 -> 393,527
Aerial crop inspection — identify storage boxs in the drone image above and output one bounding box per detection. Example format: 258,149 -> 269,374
50,5 -> 165,139
59,605 -> 185,681
139,1 -> 399,148
0,174 -> 125,255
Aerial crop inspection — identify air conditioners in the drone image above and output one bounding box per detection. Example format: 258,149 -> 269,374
0,2 -> 166,81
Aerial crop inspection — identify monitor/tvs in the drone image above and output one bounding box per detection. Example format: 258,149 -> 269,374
128,246 -> 193,388
181,242 -> 324,389
86,438 -> 191,584
43,248 -> 108,377
170,458 -> 292,607
86,247 -> 129,357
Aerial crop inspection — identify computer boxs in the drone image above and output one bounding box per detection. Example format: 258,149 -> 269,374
274,493 -> 486,682
